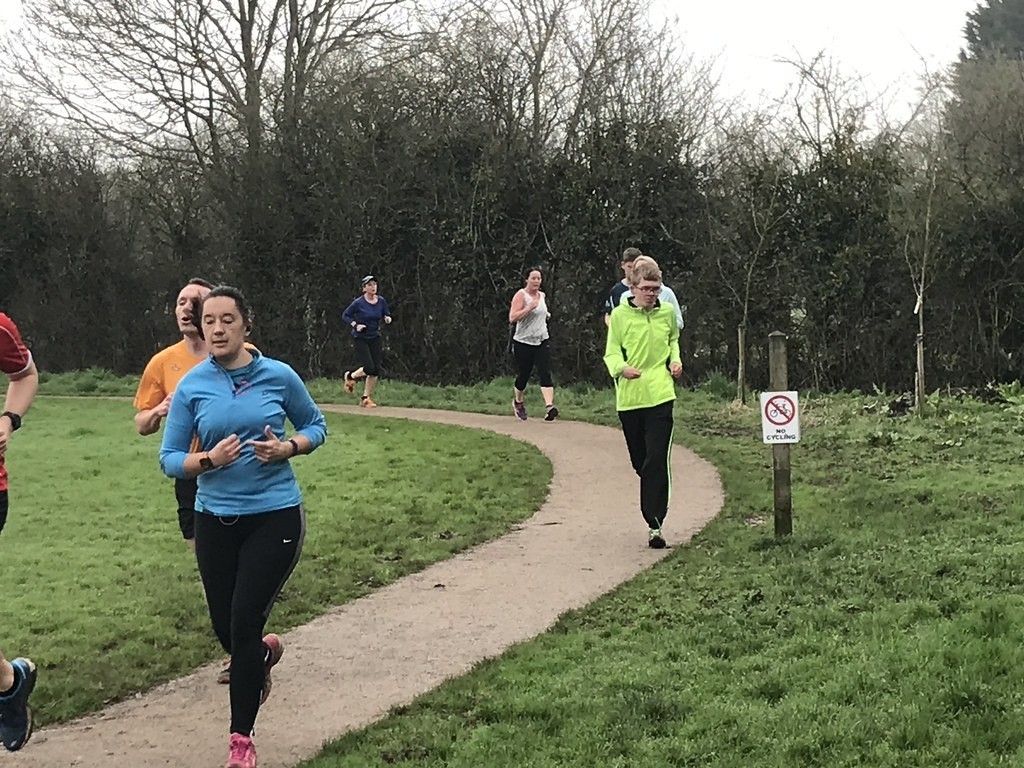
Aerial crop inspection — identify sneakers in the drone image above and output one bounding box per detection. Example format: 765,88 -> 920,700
259,633 -> 284,705
360,396 -> 377,408
545,406 -> 559,421
0,656 -> 37,751
648,517 -> 666,548
344,371 -> 356,393
512,399 -> 527,421
225,732 -> 257,768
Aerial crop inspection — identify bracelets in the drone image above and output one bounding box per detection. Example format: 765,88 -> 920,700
287,438 -> 299,456
353,323 -> 359,328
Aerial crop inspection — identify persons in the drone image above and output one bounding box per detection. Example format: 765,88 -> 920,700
158,286 -> 328,768
342,275 -> 392,408
0,313 -> 39,752
134,278 -> 264,683
605,248 -> 683,392
604,261 -> 683,547
508,266 -> 559,421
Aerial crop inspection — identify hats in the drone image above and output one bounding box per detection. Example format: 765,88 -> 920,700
362,275 -> 378,287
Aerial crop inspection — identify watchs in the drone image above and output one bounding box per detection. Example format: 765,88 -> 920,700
198,451 -> 214,471
2,411 -> 22,431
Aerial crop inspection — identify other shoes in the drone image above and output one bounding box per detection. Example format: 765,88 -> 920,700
216,655 -> 232,683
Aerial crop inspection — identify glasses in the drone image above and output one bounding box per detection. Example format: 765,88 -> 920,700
635,286 -> 663,294
